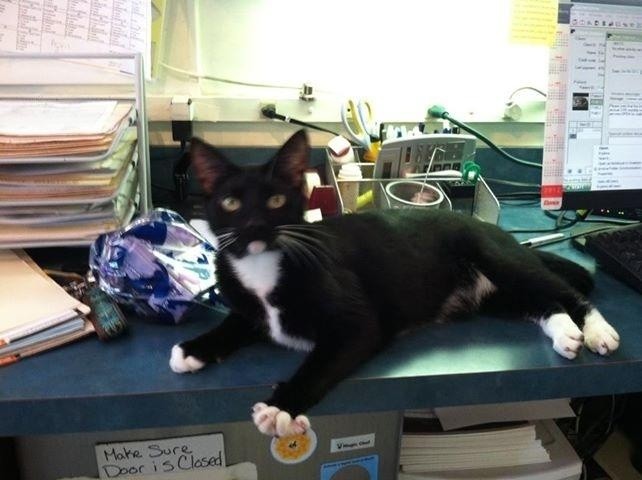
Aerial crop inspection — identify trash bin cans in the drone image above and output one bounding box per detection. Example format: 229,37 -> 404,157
520,232 -> 567,249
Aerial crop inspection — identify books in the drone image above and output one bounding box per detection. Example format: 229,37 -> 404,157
1,249 -> 96,365
0,98 -> 140,252
398,397 -> 572,475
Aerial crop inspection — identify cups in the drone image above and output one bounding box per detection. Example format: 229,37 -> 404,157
386,180 -> 444,211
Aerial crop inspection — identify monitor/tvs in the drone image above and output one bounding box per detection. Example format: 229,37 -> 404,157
540,0 -> 642,208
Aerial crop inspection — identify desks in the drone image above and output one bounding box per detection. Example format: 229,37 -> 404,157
1,145 -> 642,479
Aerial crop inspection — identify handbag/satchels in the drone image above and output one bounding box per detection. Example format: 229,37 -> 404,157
88,207 -> 231,327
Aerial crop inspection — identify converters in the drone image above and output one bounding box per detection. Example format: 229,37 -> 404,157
171,120 -> 192,141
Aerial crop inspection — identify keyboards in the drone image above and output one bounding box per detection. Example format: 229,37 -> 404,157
585,223 -> 642,293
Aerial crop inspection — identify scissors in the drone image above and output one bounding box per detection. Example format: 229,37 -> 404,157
343,98 -> 372,149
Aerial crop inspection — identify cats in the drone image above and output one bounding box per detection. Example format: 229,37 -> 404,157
168,128 -> 621,439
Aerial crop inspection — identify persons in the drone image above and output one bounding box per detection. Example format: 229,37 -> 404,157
574,98 -> 588,108
